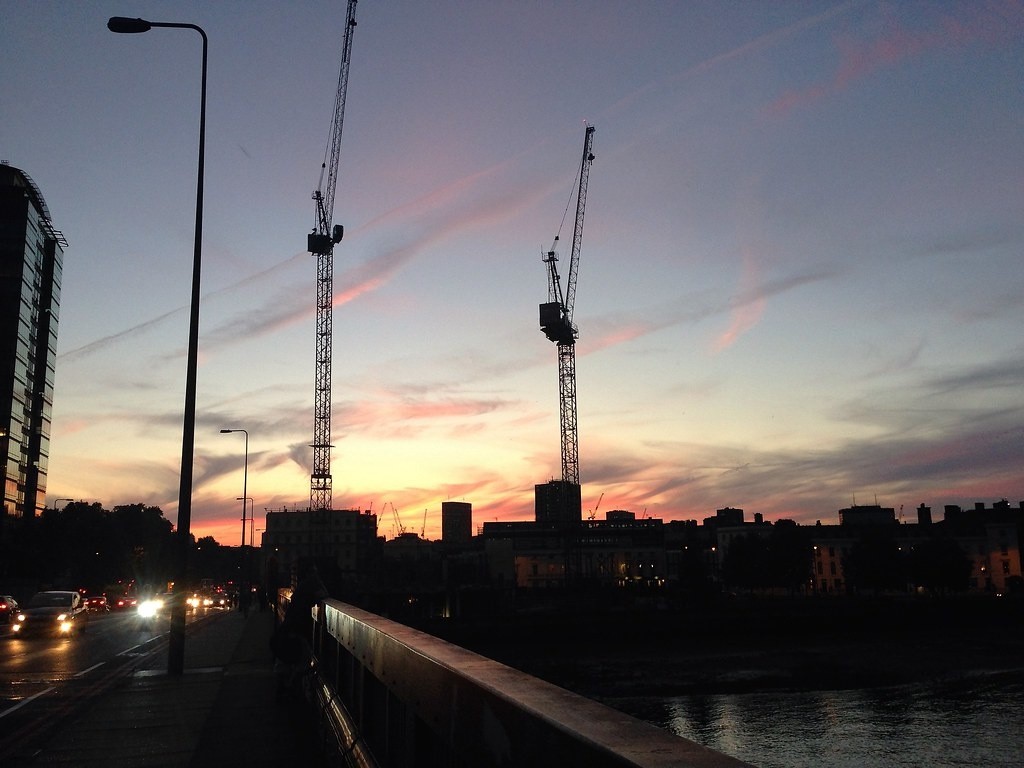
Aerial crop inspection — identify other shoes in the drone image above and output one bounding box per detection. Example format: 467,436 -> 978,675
295,687 -> 307,706
245,615 -> 248,620
238,609 -> 243,612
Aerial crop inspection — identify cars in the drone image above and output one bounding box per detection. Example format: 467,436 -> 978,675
13,590 -> 89,638
0,597 -> 19,625
79,578 -> 232,615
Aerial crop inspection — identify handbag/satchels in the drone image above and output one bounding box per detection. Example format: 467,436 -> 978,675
269,633 -> 311,667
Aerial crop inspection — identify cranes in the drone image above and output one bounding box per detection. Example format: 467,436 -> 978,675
308,0 -> 358,512
537,117 -> 596,485
369,502 -> 428,539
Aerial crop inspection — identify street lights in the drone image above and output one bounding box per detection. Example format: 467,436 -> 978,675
220,429 -> 255,546
106,17 -> 212,687
